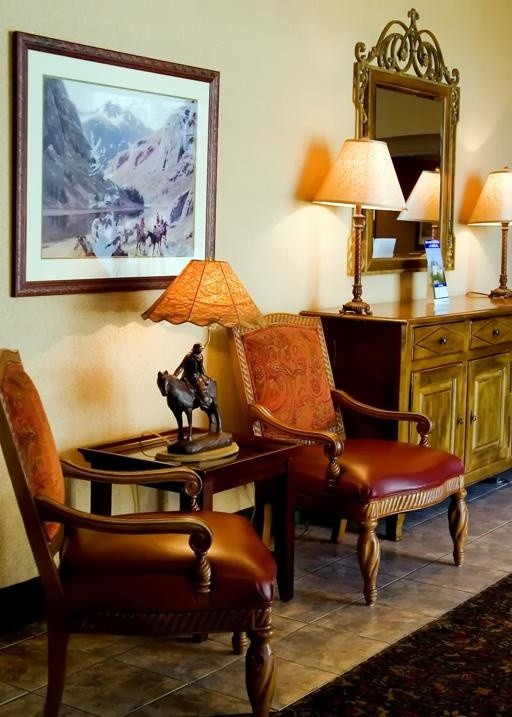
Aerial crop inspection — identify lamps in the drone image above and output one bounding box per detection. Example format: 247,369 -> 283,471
141,258 -> 268,379
396,167 -> 441,240
311,135 -> 408,316
467,166 -> 512,300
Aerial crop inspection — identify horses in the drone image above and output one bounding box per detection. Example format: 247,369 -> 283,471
147,231 -> 163,257
133,222 -> 146,255
158,222 -> 169,248
72,233 -> 128,256
156,370 -> 222,446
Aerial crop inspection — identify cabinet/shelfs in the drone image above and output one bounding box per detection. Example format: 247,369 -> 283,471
299,292 -> 512,541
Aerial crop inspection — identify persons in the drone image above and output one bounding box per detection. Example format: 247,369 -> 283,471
72,209 -> 174,257
175,342 -> 214,408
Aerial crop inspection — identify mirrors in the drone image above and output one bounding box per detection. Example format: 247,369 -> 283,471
346,7 -> 460,277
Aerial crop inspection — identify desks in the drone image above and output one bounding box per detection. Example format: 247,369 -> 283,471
76,424 -> 305,604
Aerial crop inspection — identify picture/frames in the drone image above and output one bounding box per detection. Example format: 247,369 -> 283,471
12,30 -> 219,296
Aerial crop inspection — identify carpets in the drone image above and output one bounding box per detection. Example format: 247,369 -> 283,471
260,573 -> 512,717
348,468 -> 512,534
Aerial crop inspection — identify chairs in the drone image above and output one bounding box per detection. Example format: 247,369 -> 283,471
0,348 -> 278,717
231,313 -> 467,606
